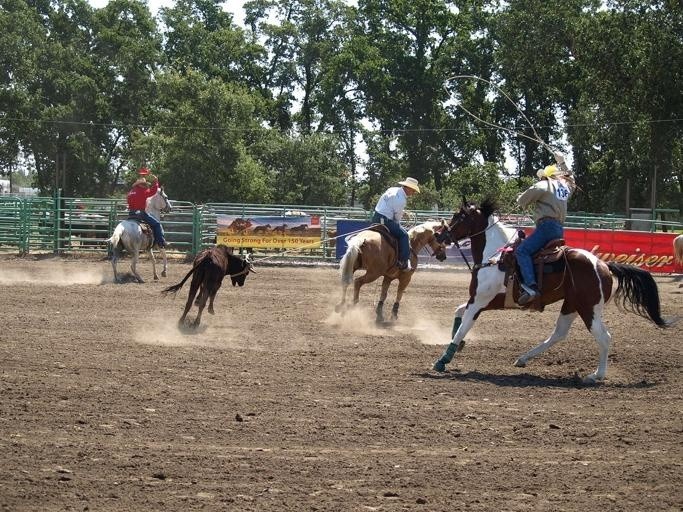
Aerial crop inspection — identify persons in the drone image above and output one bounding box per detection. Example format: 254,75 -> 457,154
515,151 -> 576,304
126,176 -> 167,248
373,176 -> 422,273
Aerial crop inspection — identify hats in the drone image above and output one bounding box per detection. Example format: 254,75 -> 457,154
130,177 -> 152,188
534,164 -> 562,180
396,176 -> 421,195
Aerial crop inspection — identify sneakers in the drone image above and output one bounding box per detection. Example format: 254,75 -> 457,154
516,283 -> 543,306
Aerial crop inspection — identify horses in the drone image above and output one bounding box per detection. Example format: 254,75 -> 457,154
253,223 -> 271,234
433,193 -> 679,385
334,215 -> 453,325
291,224 -> 308,236
673,235 -> 683,266
273,224 -> 288,237
102,185 -> 172,283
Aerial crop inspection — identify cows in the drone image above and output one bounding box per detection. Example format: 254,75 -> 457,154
160,244 -> 257,328
